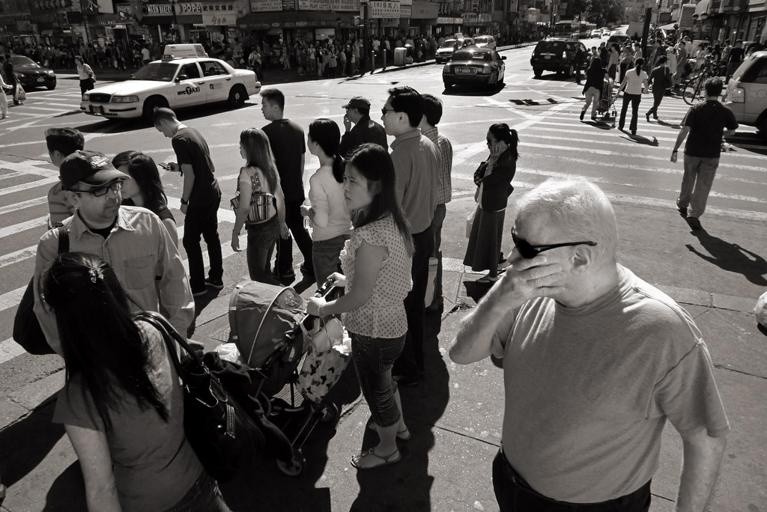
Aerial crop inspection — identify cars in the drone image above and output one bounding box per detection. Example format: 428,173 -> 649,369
435,33 -> 506,91
79,41 -> 262,127
0,54 -> 57,92
529,19 -> 612,81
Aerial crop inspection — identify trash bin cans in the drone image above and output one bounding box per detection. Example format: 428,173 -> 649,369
393,47 -> 407,66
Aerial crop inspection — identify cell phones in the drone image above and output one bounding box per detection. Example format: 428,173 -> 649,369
496,140 -> 507,154
159,162 -> 168,169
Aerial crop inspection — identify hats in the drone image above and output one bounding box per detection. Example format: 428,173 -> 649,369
58,149 -> 131,186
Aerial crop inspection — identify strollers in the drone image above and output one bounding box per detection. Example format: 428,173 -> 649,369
188,276 -> 344,484
590,81 -> 617,122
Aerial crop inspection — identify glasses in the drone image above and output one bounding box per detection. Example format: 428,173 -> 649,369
62,180 -> 124,199
381,107 -> 395,117
510,226 -> 597,261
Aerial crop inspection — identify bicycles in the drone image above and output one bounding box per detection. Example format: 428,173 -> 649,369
681,53 -> 717,106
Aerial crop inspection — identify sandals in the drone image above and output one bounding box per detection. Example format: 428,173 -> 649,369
367,418 -> 417,444
350,446 -> 402,470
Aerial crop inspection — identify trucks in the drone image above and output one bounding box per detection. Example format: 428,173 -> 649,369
721,50 -> 766,139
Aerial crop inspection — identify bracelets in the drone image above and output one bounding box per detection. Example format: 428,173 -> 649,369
672,150 -> 677,153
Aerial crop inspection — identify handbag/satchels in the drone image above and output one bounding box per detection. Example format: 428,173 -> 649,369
183,363 -> 251,446
12,274 -> 58,357
465,206 -> 479,240
228,191 -> 280,227
294,341 -> 354,407
88,73 -> 97,82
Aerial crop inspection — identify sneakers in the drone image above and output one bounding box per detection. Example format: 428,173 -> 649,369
644,111 -> 659,123
689,216 -> 699,225
191,287 -> 208,297
204,277 -> 226,290
579,110 -> 586,121
341,97 -> 372,113
301,261 -> 313,276
477,275 -> 500,284
616,124 -> 639,134
675,196 -> 688,212
273,265 -> 296,278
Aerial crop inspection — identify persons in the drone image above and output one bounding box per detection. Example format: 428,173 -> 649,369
447,178 -> 727,511
578,22 -> 757,135
669,77 -> 738,225
462,126 -> 520,284
0,71 -> 13,120
